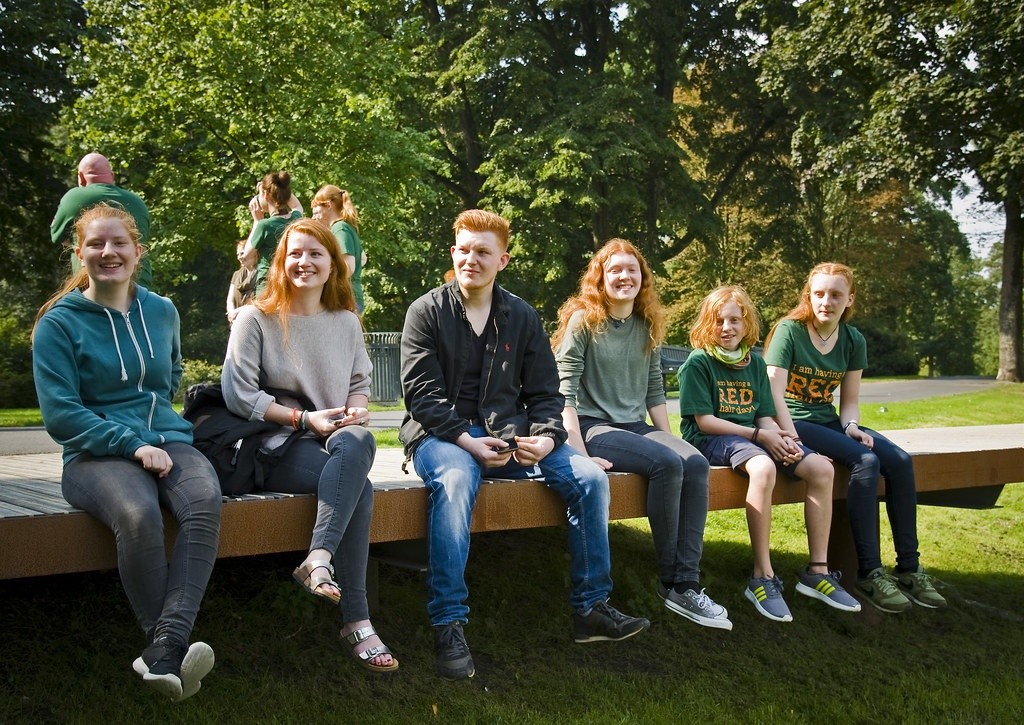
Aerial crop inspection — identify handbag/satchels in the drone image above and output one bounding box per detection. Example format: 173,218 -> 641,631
178,379 -> 316,495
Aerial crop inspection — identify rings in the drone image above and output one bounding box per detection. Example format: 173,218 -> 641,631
361,418 -> 365,425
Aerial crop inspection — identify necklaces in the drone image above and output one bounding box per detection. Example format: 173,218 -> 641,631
608,311 -> 633,323
812,319 -> 838,346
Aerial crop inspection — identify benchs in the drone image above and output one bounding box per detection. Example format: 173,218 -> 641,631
0,426 -> 1024,579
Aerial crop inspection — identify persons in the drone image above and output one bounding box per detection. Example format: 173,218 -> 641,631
762,262 -> 947,613
226,170 -> 368,326
399,209 -> 650,683
50,153 -> 153,291
549,239 -> 734,631
675,285 -> 861,621
31,201 -> 223,703
220,218 -> 399,673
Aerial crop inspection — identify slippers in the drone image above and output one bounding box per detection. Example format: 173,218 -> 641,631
339,622 -> 399,672
292,561 -> 343,605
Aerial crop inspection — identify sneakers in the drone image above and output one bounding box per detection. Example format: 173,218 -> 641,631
663,586 -> 734,630
891,566 -> 947,609
432,622 -> 475,682
795,568 -> 862,612
571,600 -> 649,644
132,642 -> 184,702
657,578 -> 728,622
853,564 -> 912,613
179,640 -> 214,702
745,571 -> 793,620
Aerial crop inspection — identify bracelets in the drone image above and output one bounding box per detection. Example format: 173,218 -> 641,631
752,428 -> 759,443
792,437 -> 802,443
292,407 -> 308,431
844,421 -> 858,433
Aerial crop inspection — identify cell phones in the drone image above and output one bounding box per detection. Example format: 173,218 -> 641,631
494,438 -> 519,454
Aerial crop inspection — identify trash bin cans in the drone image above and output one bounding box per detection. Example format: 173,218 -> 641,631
364,332 -> 403,402
